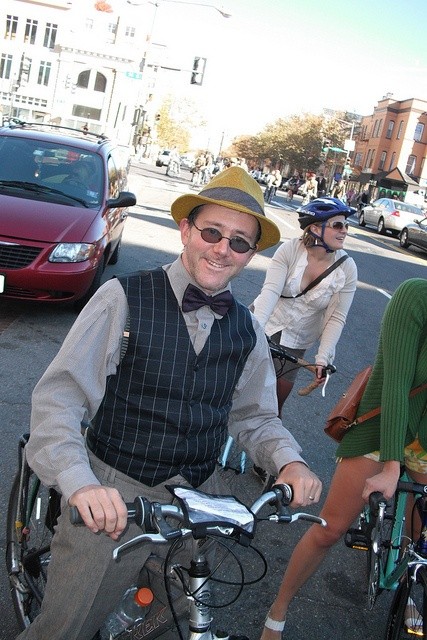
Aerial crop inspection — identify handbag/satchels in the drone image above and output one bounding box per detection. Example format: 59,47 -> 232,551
323,367 -> 425,442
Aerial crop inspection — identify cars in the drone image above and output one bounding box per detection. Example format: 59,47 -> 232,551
156,150 -> 171,166
0,123 -> 136,308
400,217 -> 427,251
359,198 -> 426,237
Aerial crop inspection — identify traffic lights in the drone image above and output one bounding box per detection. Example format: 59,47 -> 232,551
155,114 -> 160,120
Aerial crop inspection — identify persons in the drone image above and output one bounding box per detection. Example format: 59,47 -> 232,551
382,188 -> 385,191
61,161 -> 99,192
330,178 -> 346,199
259,276 -> 427,638
265,169 -> 275,189
315,172 -> 327,197
268,170 -> 282,196
357,190 -> 371,210
391,191 -> 401,202
248,196 -> 358,485
373,190 -> 387,201
287,171 -> 301,195
9,165 -> 322,639
252,166 -> 261,179
191,149 -> 249,187
300,172 -> 318,198
165,145 -> 184,177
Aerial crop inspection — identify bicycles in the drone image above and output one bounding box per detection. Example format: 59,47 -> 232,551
345,466 -> 427,640
190,169 -> 201,187
286,185 -> 296,202
217,333 -> 337,509
6,433 -> 327,640
301,192 -> 314,207
267,183 -> 278,204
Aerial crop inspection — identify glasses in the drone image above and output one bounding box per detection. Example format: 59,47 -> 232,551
191,219 -> 257,253
318,222 -> 349,233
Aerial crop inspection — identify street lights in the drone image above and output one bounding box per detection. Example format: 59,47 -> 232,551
324,114 -> 354,181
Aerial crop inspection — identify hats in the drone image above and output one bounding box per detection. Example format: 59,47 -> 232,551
171,166 -> 280,254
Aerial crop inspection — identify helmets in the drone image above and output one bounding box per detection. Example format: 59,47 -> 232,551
295,196 -> 357,231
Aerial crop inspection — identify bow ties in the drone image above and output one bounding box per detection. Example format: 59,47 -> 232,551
181,283 -> 234,317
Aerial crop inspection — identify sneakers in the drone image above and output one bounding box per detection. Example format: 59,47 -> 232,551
252,463 -> 278,490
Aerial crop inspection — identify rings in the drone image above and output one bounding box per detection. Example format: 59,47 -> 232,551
309,495 -> 315,502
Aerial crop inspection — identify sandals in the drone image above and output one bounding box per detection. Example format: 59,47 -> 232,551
405,596 -> 424,632
260,601 -> 287,640
